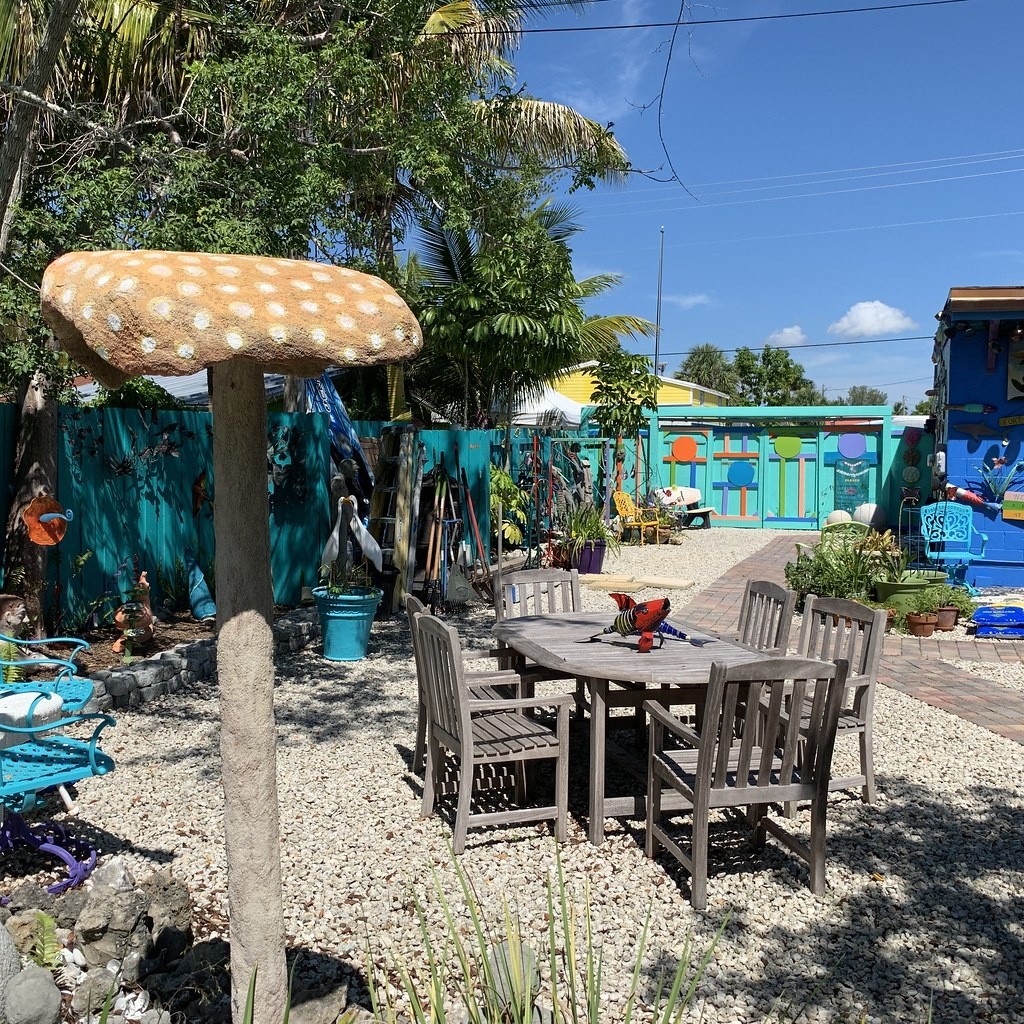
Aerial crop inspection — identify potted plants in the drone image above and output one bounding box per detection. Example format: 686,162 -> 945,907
310,555 -> 385,662
817,548 -> 976,637
622,496 -> 677,544
549,496 -> 608,575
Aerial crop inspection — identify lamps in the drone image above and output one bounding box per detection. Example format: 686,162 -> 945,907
922,419 -> 935,435
944,321 -> 988,339
1009,319 -> 1023,341
934,445 -> 946,478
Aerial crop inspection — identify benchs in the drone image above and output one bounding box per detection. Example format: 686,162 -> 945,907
654,485 -> 715,530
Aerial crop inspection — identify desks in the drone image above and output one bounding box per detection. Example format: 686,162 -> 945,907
491,609 -> 797,846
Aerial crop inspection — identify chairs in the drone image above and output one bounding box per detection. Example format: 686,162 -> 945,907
613,491 -> 661,546
921,501 -> 988,597
0,632 -> 116,906
402,567 -> 889,911
794,522 -> 871,563
934,311 -> 943,321
